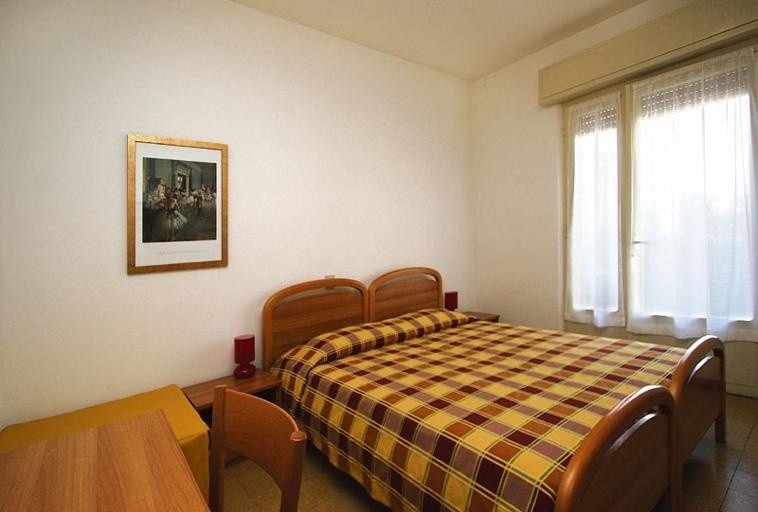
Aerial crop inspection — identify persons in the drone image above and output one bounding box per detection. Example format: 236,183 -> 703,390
146,179 -> 214,239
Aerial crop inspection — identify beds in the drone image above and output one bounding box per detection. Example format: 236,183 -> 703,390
368,266 -> 728,512
265,276 -> 679,512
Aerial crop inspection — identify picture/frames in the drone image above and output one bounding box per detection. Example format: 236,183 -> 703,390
127,132 -> 228,275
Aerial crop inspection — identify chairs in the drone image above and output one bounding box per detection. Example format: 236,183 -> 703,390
211,384 -> 307,511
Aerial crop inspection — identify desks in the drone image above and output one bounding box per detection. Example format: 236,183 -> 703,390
0,408 -> 212,511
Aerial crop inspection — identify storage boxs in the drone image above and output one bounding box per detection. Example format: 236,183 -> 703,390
0,384 -> 211,511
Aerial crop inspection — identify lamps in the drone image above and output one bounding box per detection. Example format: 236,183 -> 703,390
234,334 -> 256,379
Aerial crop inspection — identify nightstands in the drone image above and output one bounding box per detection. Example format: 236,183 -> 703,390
180,368 -> 283,408
458,310 -> 501,325
